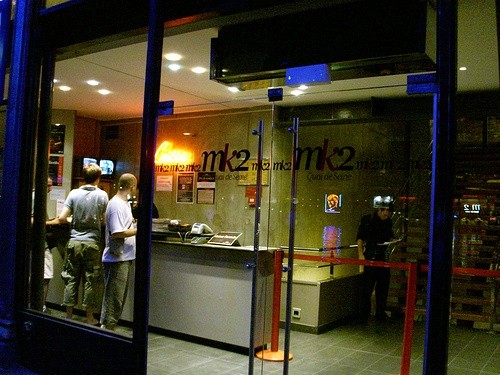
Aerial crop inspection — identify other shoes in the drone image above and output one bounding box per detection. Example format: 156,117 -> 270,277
43,308 -> 53,315
353,317 -> 367,324
94,320 -> 102,328
376,312 -> 394,324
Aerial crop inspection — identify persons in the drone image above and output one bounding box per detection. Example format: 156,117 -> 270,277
355,202 -> 395,325
131,176 -> 160,219
29,174 -> 61,315
57,163 -> 109,326
100,174 -> 137,331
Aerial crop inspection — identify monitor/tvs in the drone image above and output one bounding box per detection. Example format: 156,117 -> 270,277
81,157 -> 115,179
